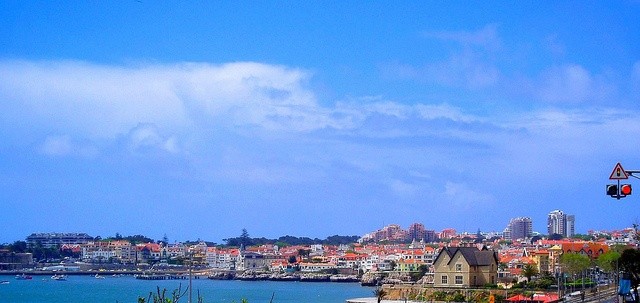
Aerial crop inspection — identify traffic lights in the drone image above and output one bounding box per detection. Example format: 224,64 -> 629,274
605,183 -> 631,194
